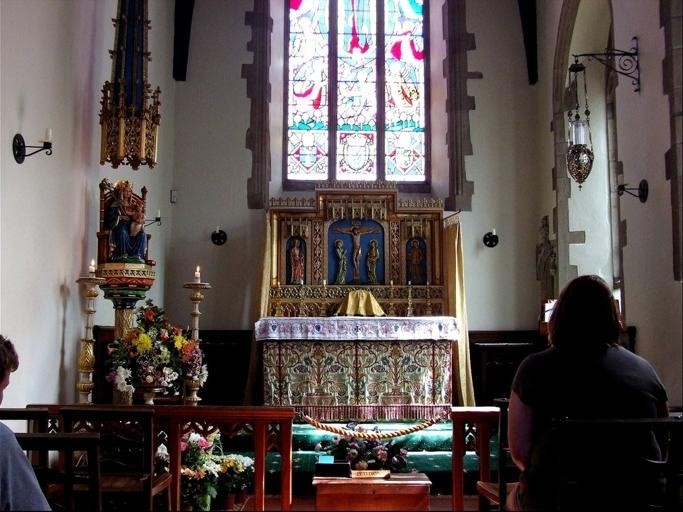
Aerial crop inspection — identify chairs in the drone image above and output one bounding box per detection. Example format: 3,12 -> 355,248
0,402 -> 177,512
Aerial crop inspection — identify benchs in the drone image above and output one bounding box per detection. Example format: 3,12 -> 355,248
471,395 -> 682,511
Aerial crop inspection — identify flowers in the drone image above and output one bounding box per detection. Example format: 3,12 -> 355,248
311,420 -> 417,475
100,297 -> 209,396
152,420 -> 255,511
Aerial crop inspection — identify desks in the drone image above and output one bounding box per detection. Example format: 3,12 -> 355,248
253,314 -> 464,422
307,468 -> 432,511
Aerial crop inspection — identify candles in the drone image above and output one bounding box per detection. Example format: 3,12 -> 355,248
214,225 -> 220,234
616,172 -> 625,186
490,227 -> 497,236
86,259 -> 97,273
154,208 -> 161,218
44,126 -> 53,143
193,265 -> 201,282
275,277 -> 431,288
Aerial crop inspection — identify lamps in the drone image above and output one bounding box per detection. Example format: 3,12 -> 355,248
561,35 -> 640,193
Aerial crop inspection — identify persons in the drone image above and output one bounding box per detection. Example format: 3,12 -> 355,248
289,238 -> 305,284
1,335 -> 53,511
477,275 -> 671,511
335,227 -> 376,277
334,239 -> 348,285
366,239 -> 380,284
104,180 -> 148,264
410,239 -> 423,284
